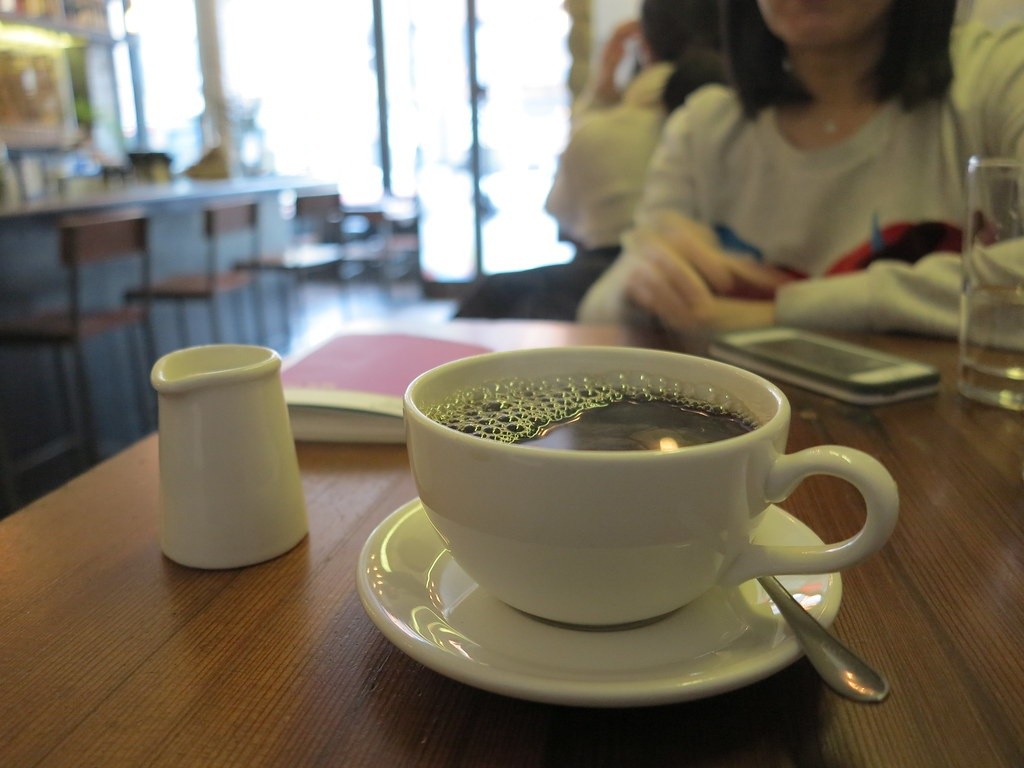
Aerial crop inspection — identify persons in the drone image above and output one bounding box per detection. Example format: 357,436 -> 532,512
542,0 -> 734,264
575,0 -> 1023,349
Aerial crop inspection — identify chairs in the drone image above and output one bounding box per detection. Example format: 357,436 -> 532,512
0,207 -> 158,464
125,199 -> 262,417
231,192 -> 342,353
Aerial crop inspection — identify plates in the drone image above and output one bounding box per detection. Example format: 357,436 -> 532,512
356,497 -> 843,710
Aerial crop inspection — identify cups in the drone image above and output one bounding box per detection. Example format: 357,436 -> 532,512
402,346 -> 899,627
151,344 -> 308,569
957,155 -> 1023,413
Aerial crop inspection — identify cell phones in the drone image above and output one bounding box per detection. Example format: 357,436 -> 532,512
707,325 -> 943,405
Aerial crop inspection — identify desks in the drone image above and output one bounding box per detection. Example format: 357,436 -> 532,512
0,314 -> 1024,768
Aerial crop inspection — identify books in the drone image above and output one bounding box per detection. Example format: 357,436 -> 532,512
278,329 -> 498,444
